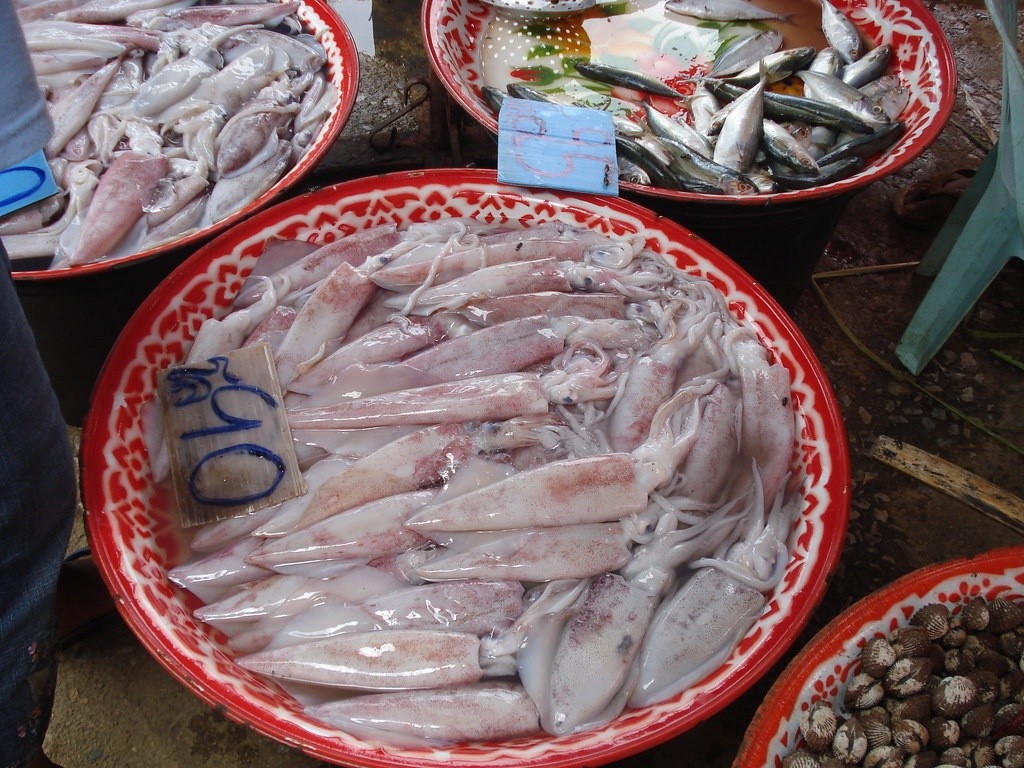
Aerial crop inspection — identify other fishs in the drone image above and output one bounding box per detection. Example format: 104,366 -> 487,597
481,1 -> 919,199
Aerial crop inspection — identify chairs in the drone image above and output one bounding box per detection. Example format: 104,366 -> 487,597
894,1 -> 1024,377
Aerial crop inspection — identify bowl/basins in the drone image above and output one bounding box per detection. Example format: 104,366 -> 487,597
421,0 -> 957,205
81,169 -> 850,768
0,0 -> 360,280
733,547 -> 1024,768
